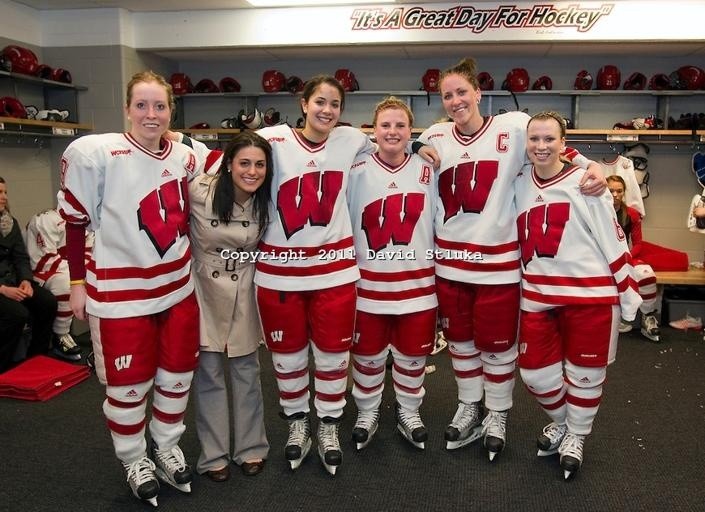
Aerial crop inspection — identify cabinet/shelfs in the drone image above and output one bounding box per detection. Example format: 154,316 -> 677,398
167,89 -> 705,146
0,70 -> 96,144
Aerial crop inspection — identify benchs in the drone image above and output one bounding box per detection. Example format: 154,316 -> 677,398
644,266 -> 704,328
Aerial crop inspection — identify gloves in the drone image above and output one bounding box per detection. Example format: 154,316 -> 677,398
623,72 -> 669,90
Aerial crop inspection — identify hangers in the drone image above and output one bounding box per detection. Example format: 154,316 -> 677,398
603,144 -> 618,162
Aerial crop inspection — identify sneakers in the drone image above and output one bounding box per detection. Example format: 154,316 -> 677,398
619,315 -> 702,336
53,334 -> 81,355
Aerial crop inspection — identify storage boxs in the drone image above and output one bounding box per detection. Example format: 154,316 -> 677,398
666,294 -> 704,329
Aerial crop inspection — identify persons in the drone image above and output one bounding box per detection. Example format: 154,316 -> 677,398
22,192 -> 94,354
0,177 -> 57,367
58,58 -> 661,501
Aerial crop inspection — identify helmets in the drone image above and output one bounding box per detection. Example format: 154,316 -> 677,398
501,68 -> 529,91
670,65 -> 705,90
422,68 -> 441,92
263,70 -> 283,92
167,73 -> 192,94
335,69 -> 357,91
597,65 -> 621,89
0,46 -> 38,78
